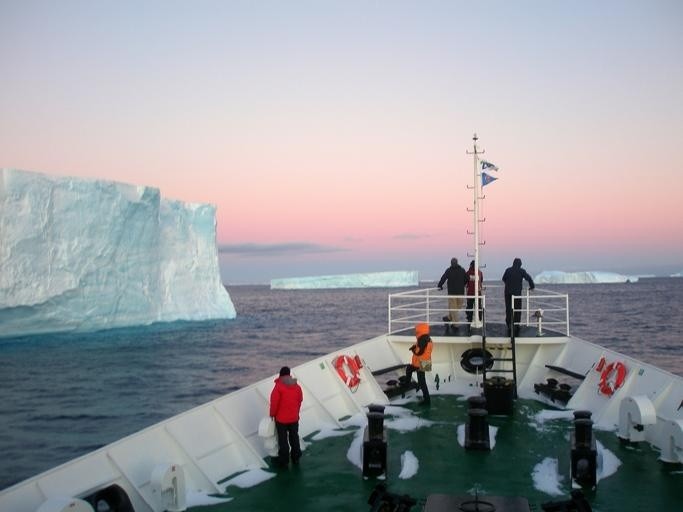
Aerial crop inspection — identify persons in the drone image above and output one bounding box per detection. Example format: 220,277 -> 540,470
399,323 -> 433,406
270,367 -> 303,464
502,258 -> 534,329
438,258 -> 470,330
467,261 -> 483,322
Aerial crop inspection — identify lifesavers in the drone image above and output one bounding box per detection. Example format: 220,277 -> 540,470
461,347 -> 494,374
598,361 -> 626,395
334,355 -> 360,387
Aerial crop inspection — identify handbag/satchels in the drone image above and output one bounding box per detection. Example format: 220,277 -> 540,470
419,360 -> 432,371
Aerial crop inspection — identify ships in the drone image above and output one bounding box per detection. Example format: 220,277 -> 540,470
0,127 -> 682,511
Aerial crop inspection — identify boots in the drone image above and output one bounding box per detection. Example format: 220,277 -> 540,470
290,443 -> 302,464
271,444 -> 289,464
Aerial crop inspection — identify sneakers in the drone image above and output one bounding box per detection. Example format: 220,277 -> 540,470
451,324 -> 459,332
443,316 -> 450,329
